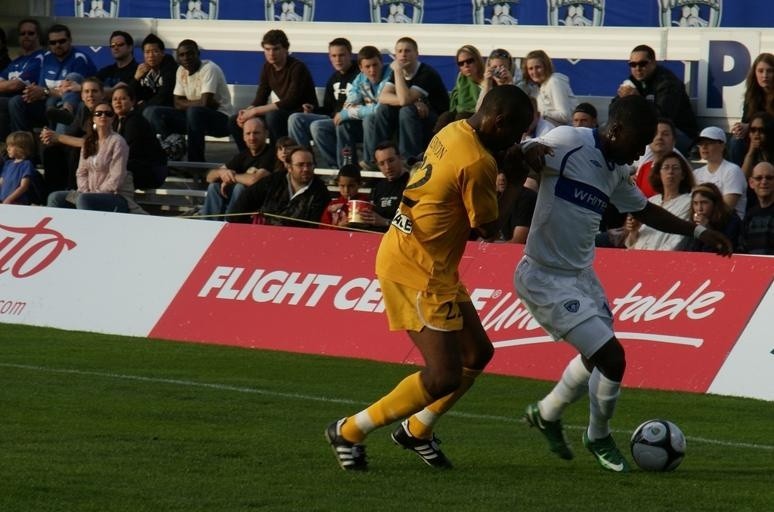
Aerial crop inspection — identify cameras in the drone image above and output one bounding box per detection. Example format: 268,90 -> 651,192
491,68 -> 502,75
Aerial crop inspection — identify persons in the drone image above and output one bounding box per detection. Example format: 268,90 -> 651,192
615,45 -> 774,254
200,29 -> 596,246
516,92 -> 737,473
0,17 -> 237,214
322,82 -> 530,473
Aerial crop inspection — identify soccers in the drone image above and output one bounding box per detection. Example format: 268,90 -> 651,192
631,420 -> 686,469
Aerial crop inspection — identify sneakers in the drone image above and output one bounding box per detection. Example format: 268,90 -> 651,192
525,402 -> 574,459
582,430 -> 632,473
390,419 -> 452,470
323,417 -> 367,472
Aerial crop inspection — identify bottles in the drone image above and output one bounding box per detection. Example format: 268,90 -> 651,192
341,145 -> 352,164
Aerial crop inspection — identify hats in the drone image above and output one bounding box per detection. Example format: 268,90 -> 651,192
700,127 -> 726,143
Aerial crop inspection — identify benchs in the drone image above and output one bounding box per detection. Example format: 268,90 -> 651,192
132,126 -> 410,206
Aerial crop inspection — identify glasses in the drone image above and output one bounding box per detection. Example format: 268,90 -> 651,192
752,176 -> 772,181
20,31 -> 35,36
457,58 -> 473,66
750,127 -> 762,133
49,39 -> 66,45
628,61 -> 647,67
93,111 -> 113,117
111,42 -> 124,47
